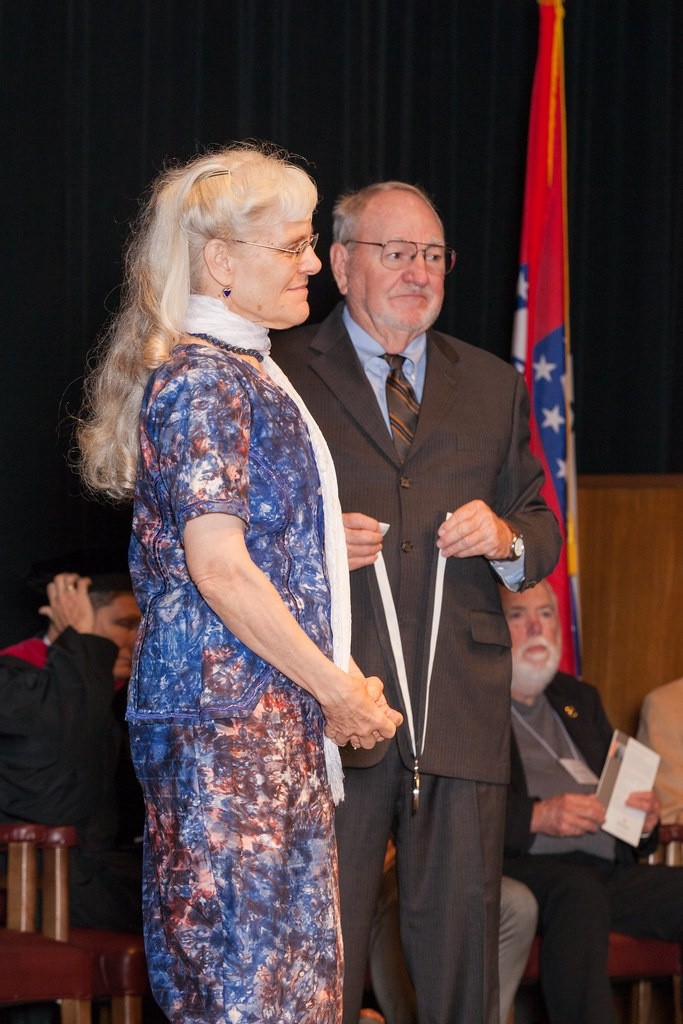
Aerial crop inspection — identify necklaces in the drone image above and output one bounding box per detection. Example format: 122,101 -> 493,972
188,333 -> 264,362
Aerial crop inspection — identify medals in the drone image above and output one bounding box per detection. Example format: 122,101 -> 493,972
412,771 -> 420,813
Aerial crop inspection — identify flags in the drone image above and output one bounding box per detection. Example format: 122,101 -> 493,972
513,0 -> 582,678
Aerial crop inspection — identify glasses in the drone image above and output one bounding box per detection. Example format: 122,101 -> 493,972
205,233 -> 320,263
343,238 -> 458,276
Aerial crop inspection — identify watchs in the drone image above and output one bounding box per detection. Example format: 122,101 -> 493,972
501,517 -> 524,562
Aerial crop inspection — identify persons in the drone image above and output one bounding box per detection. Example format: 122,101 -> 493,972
1,572 -> 171,1024
636,677 -> 683,865
500,580 -> 683,1024
271,183 -> 561,1023
369,839 -> 538,1024
81,151 -> 402,1023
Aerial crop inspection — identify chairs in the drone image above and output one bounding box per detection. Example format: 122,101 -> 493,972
521,823 -> 683,1024
0,822 -> 149,1023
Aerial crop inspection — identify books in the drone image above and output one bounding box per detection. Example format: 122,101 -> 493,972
597,729 -> 661,847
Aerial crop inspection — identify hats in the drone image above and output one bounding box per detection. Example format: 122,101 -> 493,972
24,533 -> 131,587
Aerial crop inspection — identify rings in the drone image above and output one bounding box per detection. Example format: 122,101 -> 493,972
351,741 -> 360,749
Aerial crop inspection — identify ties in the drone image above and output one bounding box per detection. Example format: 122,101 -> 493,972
379,351 -> 419,463
